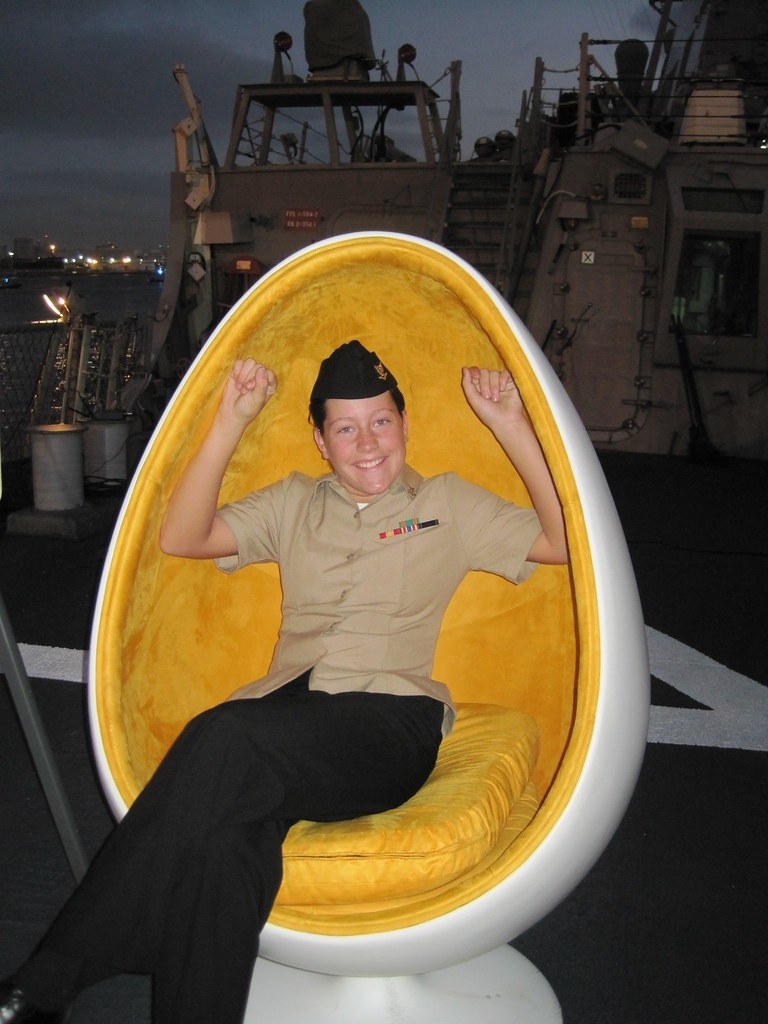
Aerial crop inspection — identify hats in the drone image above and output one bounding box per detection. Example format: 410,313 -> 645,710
310,337 -> 396,401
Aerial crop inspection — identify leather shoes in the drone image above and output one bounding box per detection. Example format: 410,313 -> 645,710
0,981 -> 73,1024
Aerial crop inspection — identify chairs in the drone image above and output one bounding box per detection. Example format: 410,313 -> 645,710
89,230 -> 650,974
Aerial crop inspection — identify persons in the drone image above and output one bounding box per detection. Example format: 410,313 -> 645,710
0,337 -> 576,1024
